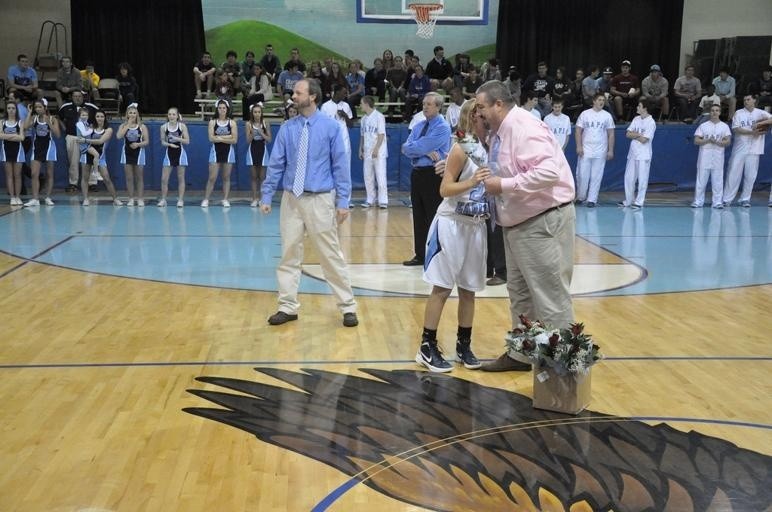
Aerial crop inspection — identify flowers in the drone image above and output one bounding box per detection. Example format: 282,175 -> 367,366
454,129 -> 505,204
504,314 -> 601,383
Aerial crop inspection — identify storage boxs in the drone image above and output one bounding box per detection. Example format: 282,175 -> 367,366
532,369 -> 591,415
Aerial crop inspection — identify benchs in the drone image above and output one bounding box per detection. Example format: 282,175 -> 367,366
194,86 -> 455,121
4,80 -> 90,101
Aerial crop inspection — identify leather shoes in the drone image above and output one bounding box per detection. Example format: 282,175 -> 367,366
89,185 -> 99,193
64,183 -> 79,193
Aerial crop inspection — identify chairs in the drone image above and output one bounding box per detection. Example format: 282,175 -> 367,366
93,78 -> 124,120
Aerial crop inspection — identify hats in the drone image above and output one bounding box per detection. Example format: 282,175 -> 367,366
648,64 -> 661,73
602,67 -> 613,74
621,59 -> 633,67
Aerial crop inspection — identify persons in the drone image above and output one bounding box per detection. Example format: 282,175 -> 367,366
712,66 -> 736,124
639,64 -> 669,125
405,56 -> 419,79
242,63 -> 272,127
610,60 -> 641,124
582,65 -> 600,110
157,107 -> 189,208
382,50 -> 395,68
219,50 -> 242,97
240,51 -> 257,96
76,108 -> 104,180
597,65 -> 617,124
408,109 -> 427,134
535,91 -> 552,120
755,65 -> 771,115
674,66 -> 701,123
24,98 -> 61,207
572,68 -> 585,123
353,59 -> 366,80
405,65 -> 431,124
116,103 -> 149,207
722,93 -> 772,207
552,66 -> 572,121
543,98 -> 570,155
260,44 -> 281,97
201,99 -> 238,207
344,62 -> 365,123
116,63 -> 138,116
213,71 -> 235,119
446,85 -> 469,132
574,93 -> 615,207
76,110 -> 123,206
522,90 -> 541,120
462,69 -> 485,101
690,104 -> 732,209
246,104 -> 272,207
503,72 -> 521,108
277,62 -> 305,108
194,52 -> 216,98
321,84 -> 353,208
388,55 -> 408,116
325,62 -> 351,102
55,90 -> 108,193
55,57 -> 82,109
481,59 -> 501,83
321,58 -> 333,76
358,96 -> 388,209
416,99 -> 490,373
365,58 -> 386,108
684,84 -> 720,153
0,101 -> 25,205
307,61 -> 330,111
6,54 -> 44,101
452,54 -> 475,88
284,48 -> 307,77
9,90 -> 46,195
524,62 -> 555,99
426,46 -> 454,89
261,78 -> 359,327
401,92 -> 452,266
616,100 -> 657,209
475,80 -> 576,372
80,64 -> 100,108
401,49 -> 414,71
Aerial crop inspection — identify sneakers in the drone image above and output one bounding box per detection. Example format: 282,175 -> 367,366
379,204 -> 387,209
574,198 -> 584,207
10,197 -> 17,205
456,339 -> 482,369
722,201 -> 732,207
15,197 -> 23,204
205,93 -> 211,98
615,202 -> 624,207
630,204 -> 643,209
83,199 -> 89,206
195,94 -> 202,99
137,199 -> 145,207
93,171 -> 103,181
113,198 -> 123,206
23,198 -> 41,206
742,201 -> 750,207
157,198 -> 167,207
221,199 -> 229,207
201,199 -> 209,207
361,201 -> 376,208
267,309 -> 298,325
712,203 -> 723,208
251,199 -> 259,207
480,350 -> 532,372
415,339 -> 454,373
586,201 -> 596,208
177,198 -> 184,208
44,197 -> 55,205
343,311 -> 358,326
690,201 -> 704,207
768,200 -> 772,206
127,198 -> 134,206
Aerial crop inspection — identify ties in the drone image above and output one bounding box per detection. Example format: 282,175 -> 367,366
411,120 -> 430,165
488,135 -> 501,232
291,119 -> 308,198
